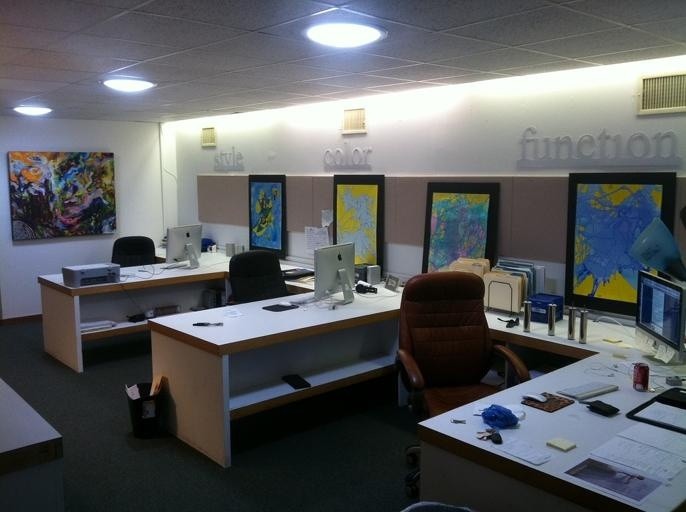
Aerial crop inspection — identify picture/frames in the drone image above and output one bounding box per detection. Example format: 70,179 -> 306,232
332,173 -> 383,275
564,172 -> 676,317
247,173 -> 285,259
419,182 -> 499,275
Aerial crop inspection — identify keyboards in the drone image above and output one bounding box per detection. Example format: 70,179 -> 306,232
556,382 -> 618,400
160,262 -> 187,269
291,295 -> 314,303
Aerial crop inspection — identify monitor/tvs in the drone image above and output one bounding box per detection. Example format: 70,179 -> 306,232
635,270 -> 686,364
314,242 -> 355,305
166,224 -> 202,269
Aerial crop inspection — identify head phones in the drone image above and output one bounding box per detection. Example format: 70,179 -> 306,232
356,284 -> 377,294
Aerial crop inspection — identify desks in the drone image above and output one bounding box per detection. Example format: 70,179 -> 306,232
146,268 -> 407,467
416,300 -> 684,512
36,243 -> 225,374
0,376 -> 67,512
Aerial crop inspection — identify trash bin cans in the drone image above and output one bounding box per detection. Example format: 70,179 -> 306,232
125,382 -> 166,440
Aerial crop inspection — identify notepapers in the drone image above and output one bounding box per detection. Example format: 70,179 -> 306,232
547,437 -> 576,452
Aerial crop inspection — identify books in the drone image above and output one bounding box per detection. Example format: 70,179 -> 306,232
449,255 -> 548,310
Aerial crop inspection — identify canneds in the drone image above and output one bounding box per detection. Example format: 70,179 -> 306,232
632,363 -> 649,392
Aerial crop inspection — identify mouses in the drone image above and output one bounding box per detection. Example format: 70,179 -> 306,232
522,393 -> 548,404
278,302 -> 291,307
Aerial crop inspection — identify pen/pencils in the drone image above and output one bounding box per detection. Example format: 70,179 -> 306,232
193,322 -> 223,326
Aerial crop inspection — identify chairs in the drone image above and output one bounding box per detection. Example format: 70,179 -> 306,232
394,269 -> 529,500
110,237 -> 155,265
229,248 -> 287,303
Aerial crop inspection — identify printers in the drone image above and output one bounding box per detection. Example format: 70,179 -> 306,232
62,262 -> 120,288
